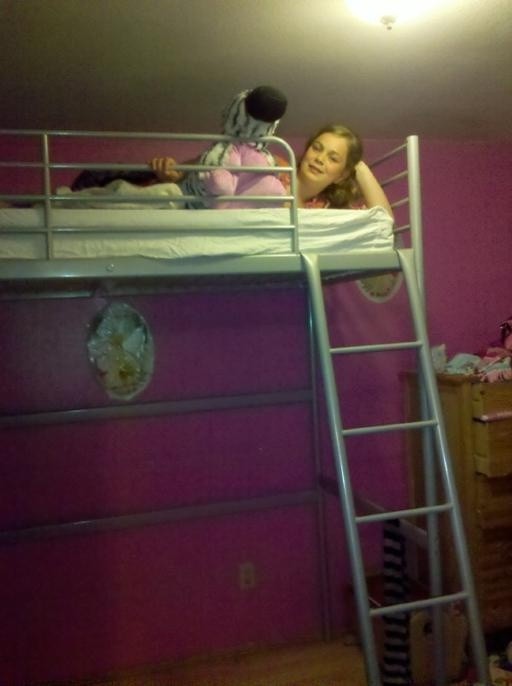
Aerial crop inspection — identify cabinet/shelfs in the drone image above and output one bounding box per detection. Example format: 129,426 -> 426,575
399,367 -> 510,634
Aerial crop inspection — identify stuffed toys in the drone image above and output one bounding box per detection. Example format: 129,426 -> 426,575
177,83 -> 288,212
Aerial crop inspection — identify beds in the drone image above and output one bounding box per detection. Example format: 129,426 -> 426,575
0,135 -> 489,686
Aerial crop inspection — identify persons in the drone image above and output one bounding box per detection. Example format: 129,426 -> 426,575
68,121 -> 393,218
